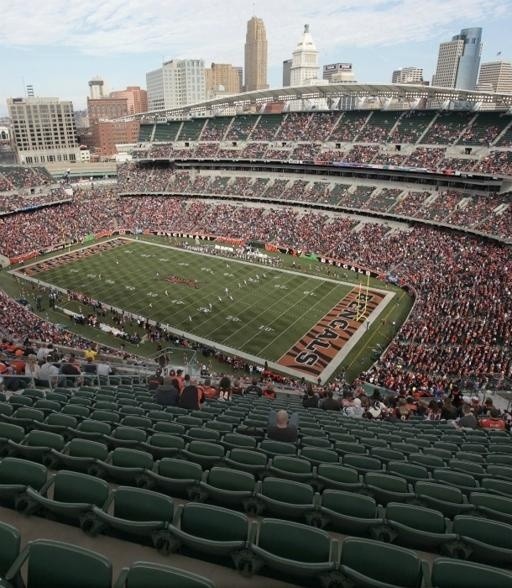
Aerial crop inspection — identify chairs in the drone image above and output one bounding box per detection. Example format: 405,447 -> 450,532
0,387 -> 511,586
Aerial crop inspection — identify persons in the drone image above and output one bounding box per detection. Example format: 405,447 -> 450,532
267,409 -> 298,443
1,109 -> 512,428
